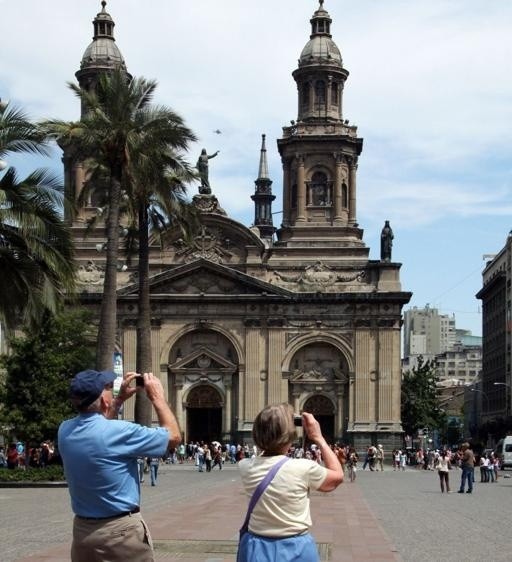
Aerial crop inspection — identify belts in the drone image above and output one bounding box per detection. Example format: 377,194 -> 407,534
76,508 -> 138,521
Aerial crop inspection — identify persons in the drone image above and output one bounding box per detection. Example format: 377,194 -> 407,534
237,403 -> 344,561
149,458 -> 160,486
381,220 -> 394,260
56,369 -> 182,562
0,440 -> 56,470
196,148 -> 220,186
457,442 -> 475,493
137,457 -> 145,483
162,442 -> 347,472
437,451 -> 453,493
363,443 -> 386,475
480,452 -> 500,483
345,447 -> 359,473
391,447 -> 464,469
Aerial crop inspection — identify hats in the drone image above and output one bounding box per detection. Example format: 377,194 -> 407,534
68,369 -> 118,401
461,442 -> 470,448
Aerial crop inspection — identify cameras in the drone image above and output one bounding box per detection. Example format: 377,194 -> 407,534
135,376 -> 145,386
293,416 -> 302,426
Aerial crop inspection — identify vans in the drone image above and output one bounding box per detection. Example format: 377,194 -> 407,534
496,434 -> 512,471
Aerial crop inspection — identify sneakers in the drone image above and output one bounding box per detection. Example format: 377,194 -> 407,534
466,490 -> 471,493
458,490 -> 464,493
